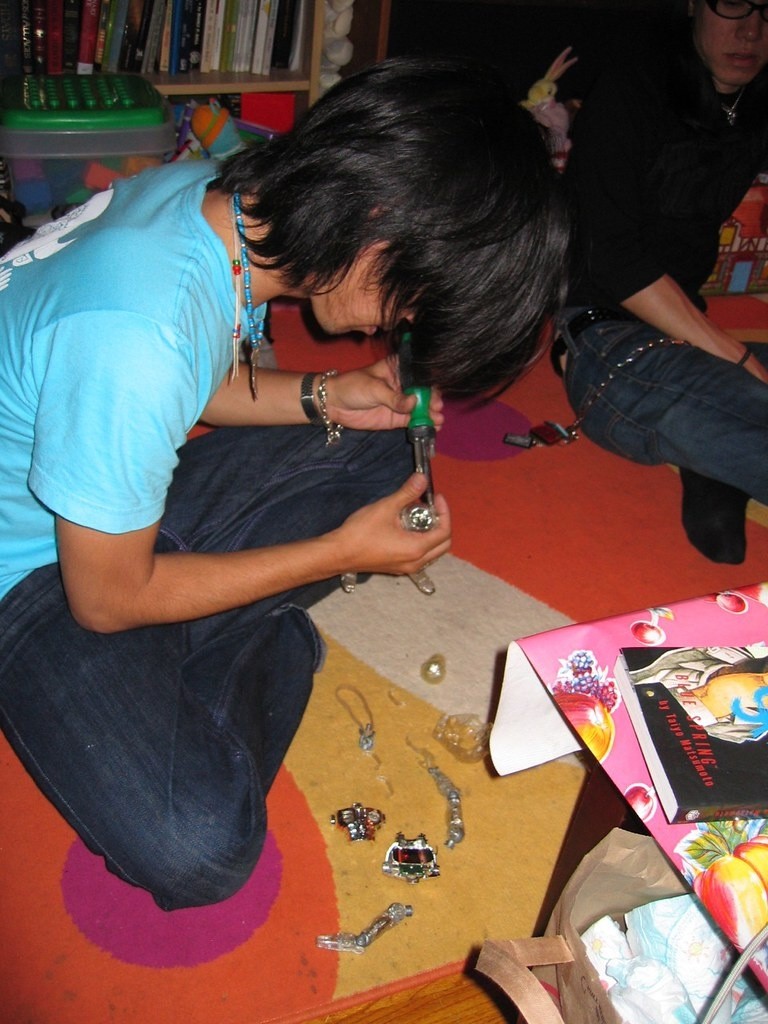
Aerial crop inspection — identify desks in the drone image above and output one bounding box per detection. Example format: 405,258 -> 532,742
489,580 -> 768,1004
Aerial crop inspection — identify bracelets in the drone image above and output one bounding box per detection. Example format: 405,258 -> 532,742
318,368 -> 344,447
301,372 -> 325,428
735,348 -> 752,369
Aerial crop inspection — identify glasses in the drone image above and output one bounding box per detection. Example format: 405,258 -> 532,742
705,0 -> 768,23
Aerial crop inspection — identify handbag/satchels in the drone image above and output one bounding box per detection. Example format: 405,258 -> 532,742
474,827 -> 768,1024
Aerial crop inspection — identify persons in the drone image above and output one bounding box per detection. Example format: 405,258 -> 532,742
0,56 -> 573,912
550,0 -> 768,565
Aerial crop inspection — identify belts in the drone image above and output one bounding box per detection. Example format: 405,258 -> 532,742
550,308 -> 641,378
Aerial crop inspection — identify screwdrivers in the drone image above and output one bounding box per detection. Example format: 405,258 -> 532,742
398,329 -> 436,509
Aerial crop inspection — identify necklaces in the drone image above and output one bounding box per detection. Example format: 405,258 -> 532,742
720,85 -> 745,127
232,191 -> 265,400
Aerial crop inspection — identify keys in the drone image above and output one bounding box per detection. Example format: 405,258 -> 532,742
191,98 -> 248,160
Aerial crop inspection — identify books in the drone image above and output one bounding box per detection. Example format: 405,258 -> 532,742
612,646 -> 767,825
0,0 -> 309,76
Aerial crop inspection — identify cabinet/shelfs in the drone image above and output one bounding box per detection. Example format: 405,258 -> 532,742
0,0 -> 392,108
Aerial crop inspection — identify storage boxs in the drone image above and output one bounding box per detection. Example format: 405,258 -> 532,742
0,74 -> 179,224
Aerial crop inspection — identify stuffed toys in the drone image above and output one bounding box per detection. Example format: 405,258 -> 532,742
519,46 -> 582,175
